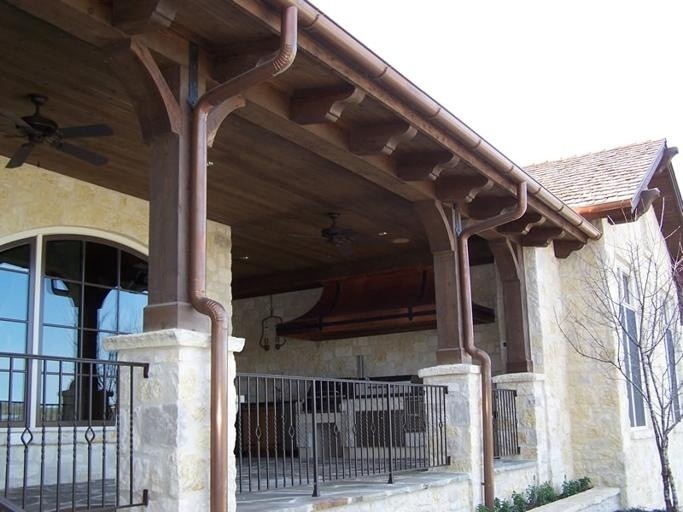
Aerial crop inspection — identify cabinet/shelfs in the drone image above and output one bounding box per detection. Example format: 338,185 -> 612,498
232,399 -> 303,457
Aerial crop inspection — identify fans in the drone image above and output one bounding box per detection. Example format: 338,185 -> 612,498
274,210 -> 414,249
1,93 -> 114,170
